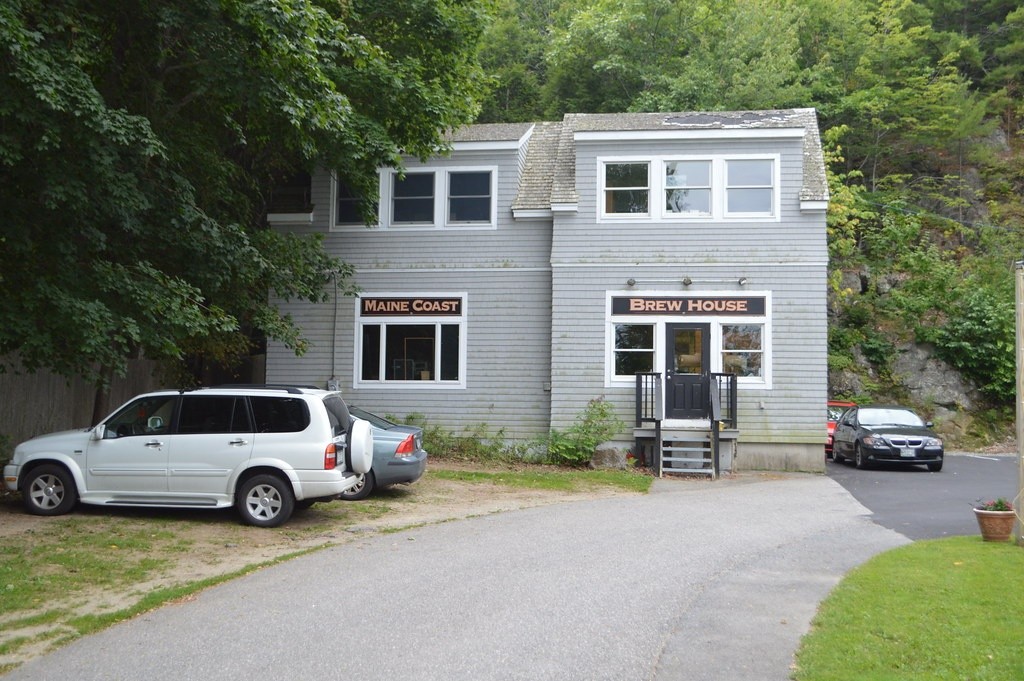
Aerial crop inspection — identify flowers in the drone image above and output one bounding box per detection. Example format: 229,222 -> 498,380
970,496 -> 1012,512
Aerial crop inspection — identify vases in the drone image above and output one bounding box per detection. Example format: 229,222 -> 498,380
973,508 -> 1016,540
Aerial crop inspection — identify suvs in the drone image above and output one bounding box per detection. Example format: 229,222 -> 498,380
3,384 -> 376,529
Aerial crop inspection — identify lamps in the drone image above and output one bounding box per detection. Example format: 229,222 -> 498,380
683,277 -> 692,285
627,279 -> 635,286
739,277 -> 746,284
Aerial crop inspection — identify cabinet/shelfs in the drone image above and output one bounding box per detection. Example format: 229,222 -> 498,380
394,359 -> 427,380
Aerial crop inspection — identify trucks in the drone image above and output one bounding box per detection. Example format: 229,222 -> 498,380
826,399 -> 855,457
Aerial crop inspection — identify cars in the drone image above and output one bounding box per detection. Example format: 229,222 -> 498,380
336,402 -> 427,503
831,404 -> 944,474
